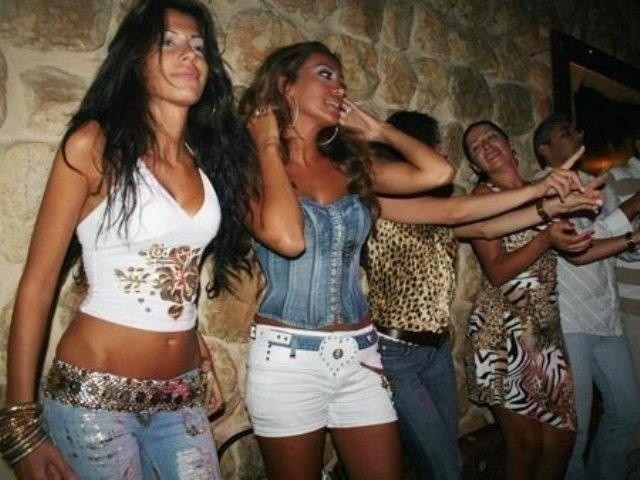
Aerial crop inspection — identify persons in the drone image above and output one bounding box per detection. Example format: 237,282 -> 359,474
597,128 -> 639,374
364,112 -> 610,475
1,0 -> 260,479
461,116 -> 640,479
237,37 -> 453,479
528,113 -> 640,479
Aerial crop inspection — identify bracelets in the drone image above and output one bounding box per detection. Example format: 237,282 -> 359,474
625,232 -> 636,253
535,199 -> 549,222
1,400 -> 50,463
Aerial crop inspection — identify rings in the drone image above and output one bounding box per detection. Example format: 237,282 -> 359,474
259,105 -> 269,115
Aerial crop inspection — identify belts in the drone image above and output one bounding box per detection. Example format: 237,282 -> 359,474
251,310 -> 371,332
375,325 -> 449,348
